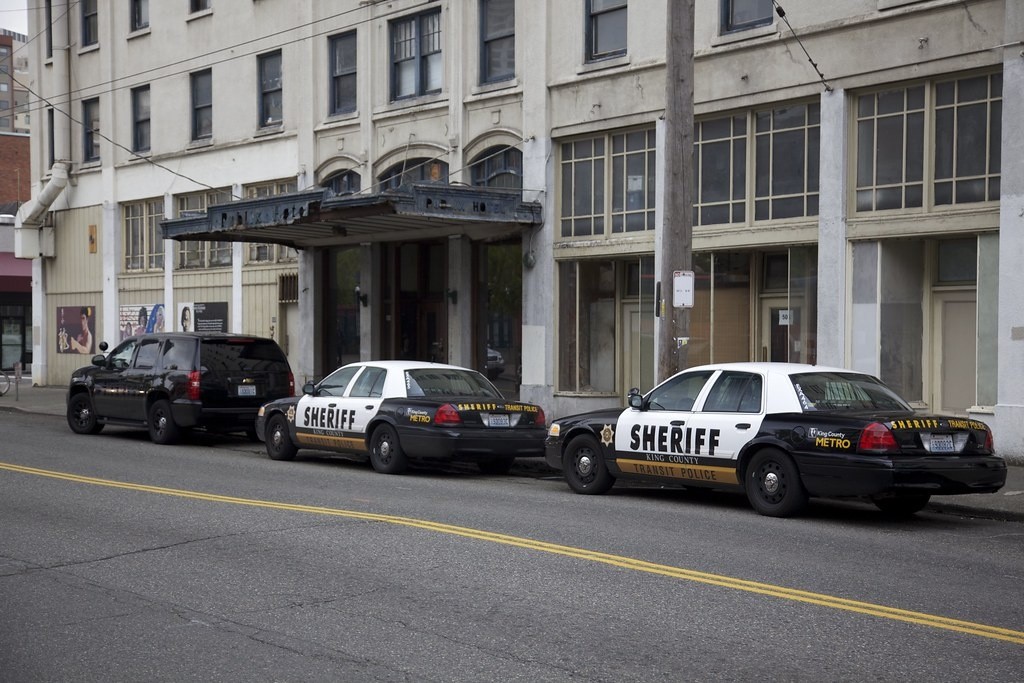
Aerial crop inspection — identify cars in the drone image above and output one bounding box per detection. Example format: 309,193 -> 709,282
254,360 -> 544,475
543,361 -> 1008,519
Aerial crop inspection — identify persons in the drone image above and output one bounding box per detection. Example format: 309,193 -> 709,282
70,307 -> 93,353
123,305 -> 164,340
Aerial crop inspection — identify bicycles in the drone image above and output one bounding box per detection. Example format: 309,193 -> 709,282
0,371 -> 10,397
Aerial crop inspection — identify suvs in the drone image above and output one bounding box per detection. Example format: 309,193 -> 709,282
66,332 -> 296,445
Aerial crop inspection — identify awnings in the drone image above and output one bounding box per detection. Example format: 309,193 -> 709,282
0,252 -> 32,292
